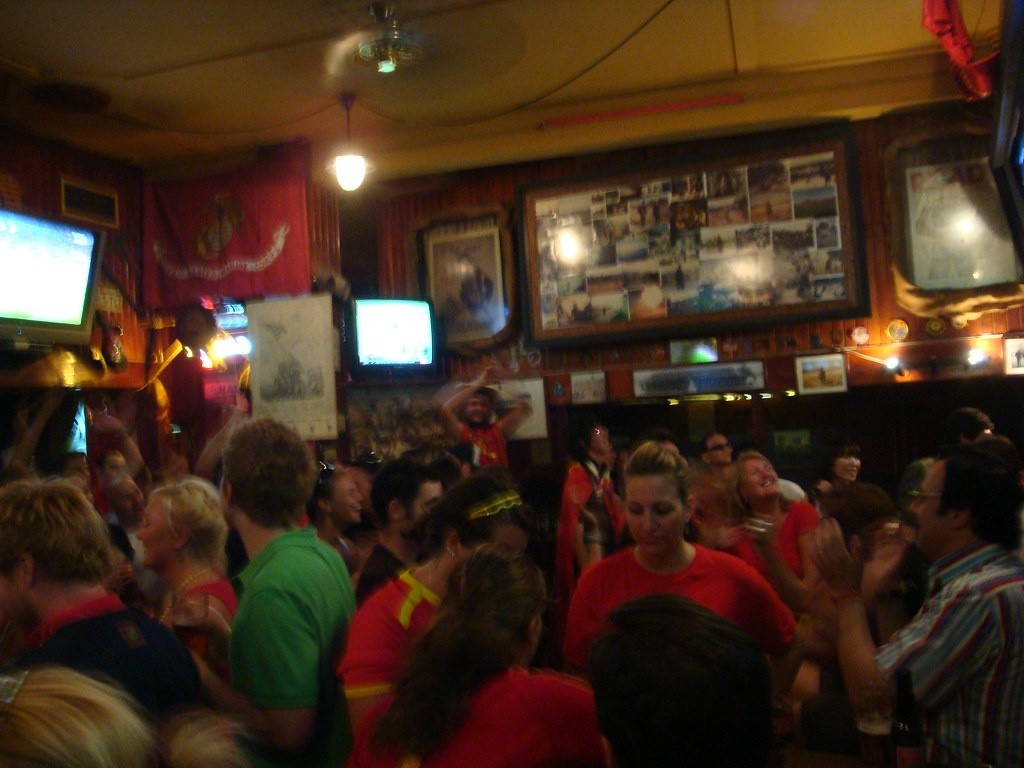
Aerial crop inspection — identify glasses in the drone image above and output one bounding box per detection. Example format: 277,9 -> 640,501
907,489 -> 942,504
707,442 -> 733,452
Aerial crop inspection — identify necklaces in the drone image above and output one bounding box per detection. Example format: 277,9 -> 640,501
159,568 -> 211,623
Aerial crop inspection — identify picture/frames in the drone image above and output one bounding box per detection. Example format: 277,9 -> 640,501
415,202 -> 520,354
900,155 -> 1024,297
1002,333 -> 1024,376
516,116 -> 871,352
792,350 -> 852,398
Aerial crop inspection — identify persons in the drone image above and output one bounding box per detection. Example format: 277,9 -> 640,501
1015,350 -> 1024,367
0,366 -> 1024,768
570,165 -> 834,321
819,367 -> 826,383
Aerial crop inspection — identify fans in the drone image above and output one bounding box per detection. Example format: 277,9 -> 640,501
265,0 -> 530,113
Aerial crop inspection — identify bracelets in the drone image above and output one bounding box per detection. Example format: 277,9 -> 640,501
585,536 -> 602,543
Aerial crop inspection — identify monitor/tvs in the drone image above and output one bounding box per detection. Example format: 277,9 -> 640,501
343,296 -> 440,378
0,209 -> 106,345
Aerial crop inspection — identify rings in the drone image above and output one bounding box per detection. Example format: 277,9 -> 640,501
818,551 -> 824,554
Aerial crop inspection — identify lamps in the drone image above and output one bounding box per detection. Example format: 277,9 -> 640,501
325,90 -> 378,192
